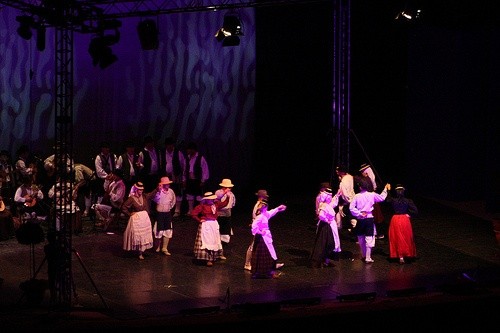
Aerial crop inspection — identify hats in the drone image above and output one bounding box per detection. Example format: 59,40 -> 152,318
52,141 -> 65,149
134,182 -> 145,189
219,178 -> 234,187
359,177 -> 373,191
159,176 -> 173,184
16,145 -> 32,152
337,161 -> 348,173
320,182 -> 330,188
254,190 -> 270,197
22,175 -> 32,184
97,135 -> 201,154
395,184 -> 406,191
358,163 -> 370,171
261,199 -> 269,205
0,150 -> 9,156
324,187 -> 332,195
111,168 -> 122,178
201,191 -> 217,199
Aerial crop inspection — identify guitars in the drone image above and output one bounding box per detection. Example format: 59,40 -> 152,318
56,181 -> 84,204
22,185 -> 42,206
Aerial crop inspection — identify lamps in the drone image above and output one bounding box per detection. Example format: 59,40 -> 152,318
394,2 -> 425,27
214,12 -> 245,46
136,18 -> 161,49
15,14 -> 38,42
87,35 -> 118,70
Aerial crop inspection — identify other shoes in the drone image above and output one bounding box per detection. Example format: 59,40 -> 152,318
162,248 -> 171,256
200,245 -> 286,280
154,243 -> 160,253
314,219 -> 413,267
138,254 -> 145,260
0,208 -> 110,236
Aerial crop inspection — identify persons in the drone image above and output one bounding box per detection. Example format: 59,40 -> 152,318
316,182 -> 343,252
213,178 -> 236,259
337,166 -> 359,243
359,163 -> 385,239
0,138 -> 210,226
251,203 -> 281,278
312,187 -> 336,265
122,181 -> 162,259
189,191 -> 230,265
386,183 -> 417,263
244,190 -> 286,269
349,181 -> 388,262
152,176 -> 176,254
